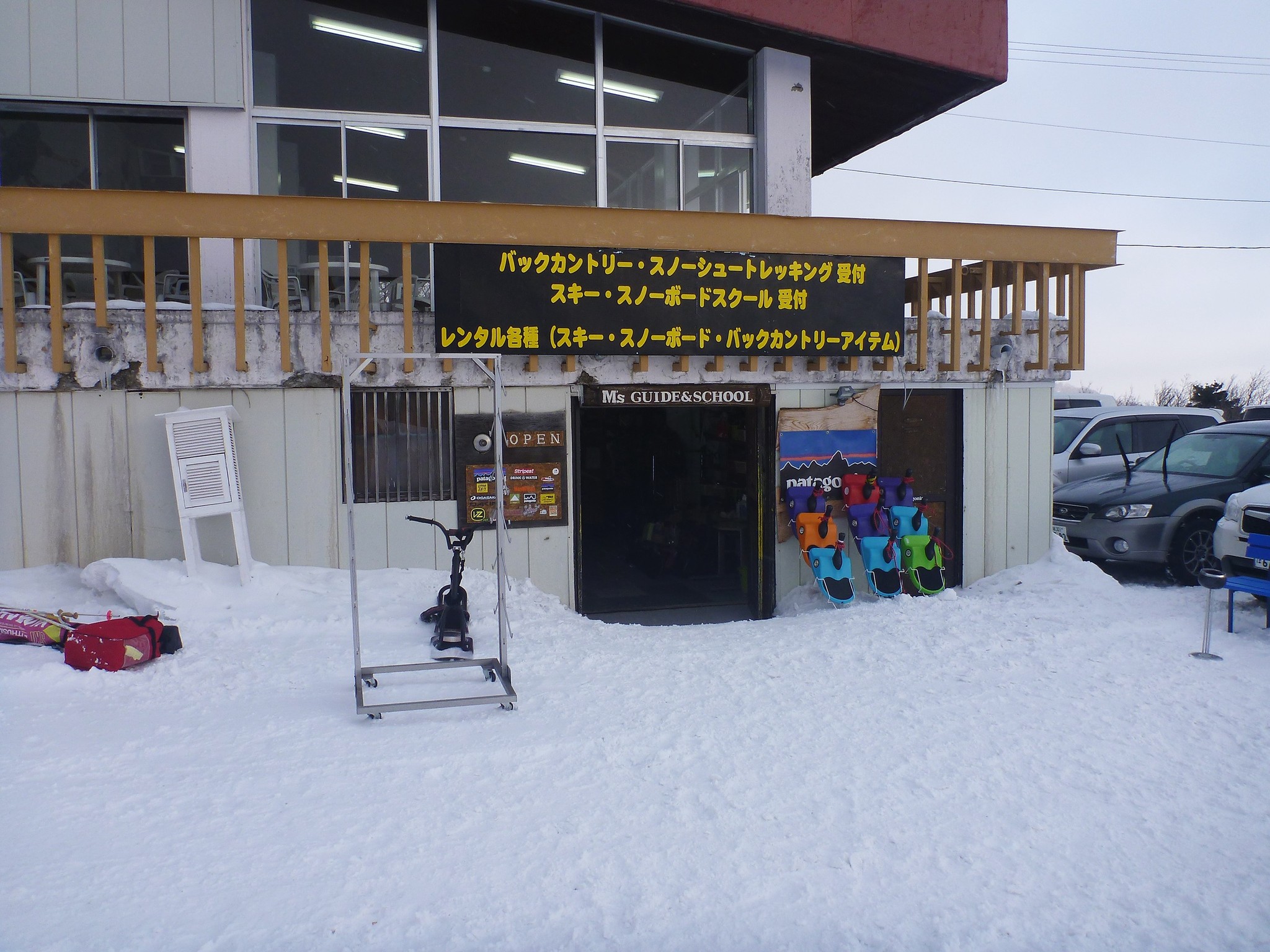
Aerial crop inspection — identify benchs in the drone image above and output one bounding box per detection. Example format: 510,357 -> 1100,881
1222,533 -> 1270,633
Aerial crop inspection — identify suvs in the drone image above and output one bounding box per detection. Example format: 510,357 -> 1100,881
1053,420 -> 1269,588
1054,392 -> 1126,453
1053,407 -> 1226,491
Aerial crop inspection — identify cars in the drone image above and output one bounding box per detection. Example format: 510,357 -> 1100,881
1213,482 -> 1270,604
1237,404 -> 1270,443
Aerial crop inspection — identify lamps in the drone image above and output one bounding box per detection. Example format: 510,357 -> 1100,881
829,386 -> 857,399
346,126 -> 410,141
555,69 -> 668,104
308,13 -> 428,54
507,150 -> 591,175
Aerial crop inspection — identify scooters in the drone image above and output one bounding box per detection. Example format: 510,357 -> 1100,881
406,514 -> 491,661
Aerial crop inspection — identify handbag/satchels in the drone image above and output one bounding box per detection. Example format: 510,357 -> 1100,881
64,615 -> 165,671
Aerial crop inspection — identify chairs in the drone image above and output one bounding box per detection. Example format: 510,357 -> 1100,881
13,266 -> 190,307
260,270 -> 432,314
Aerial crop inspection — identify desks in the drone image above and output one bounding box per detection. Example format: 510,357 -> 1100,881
297,262 -> 390,311
27,257 -> 132,305
